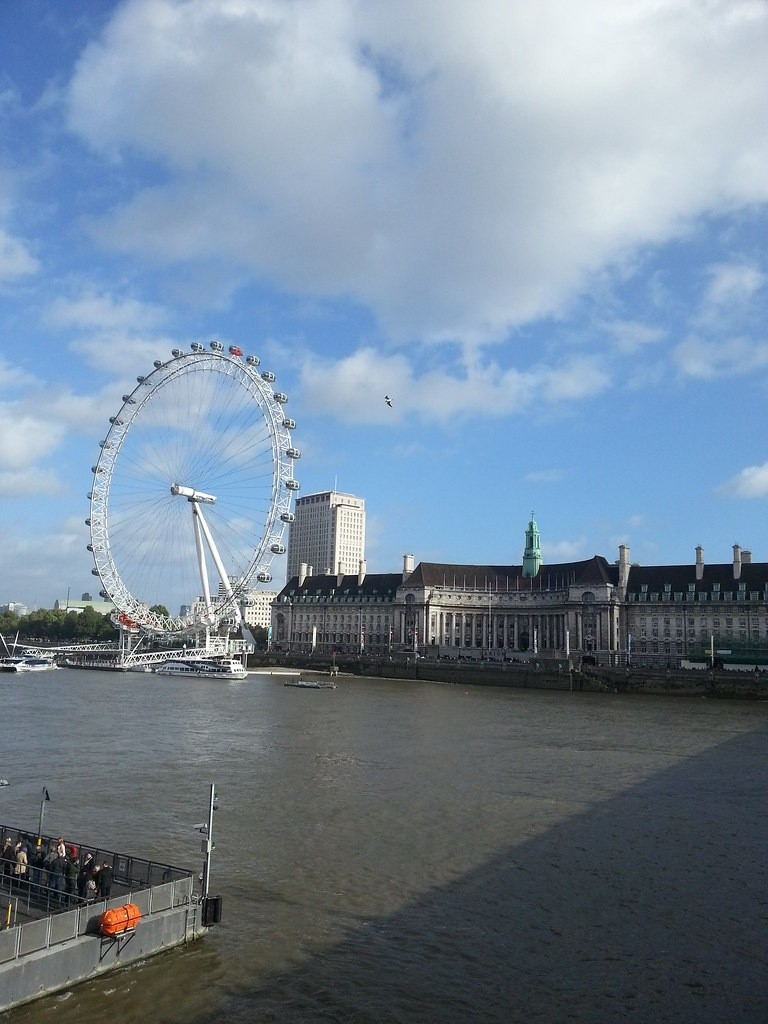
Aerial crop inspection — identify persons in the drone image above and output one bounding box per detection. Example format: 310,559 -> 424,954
1,837 -> 113,907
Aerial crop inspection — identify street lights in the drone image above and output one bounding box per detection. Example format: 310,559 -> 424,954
361,627 -> 365,655
389,626 -> 393,653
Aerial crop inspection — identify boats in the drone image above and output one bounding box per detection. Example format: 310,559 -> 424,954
0,656 -> 58,671
98,904 -> 142,934
158,658 -> 249,680
0,779 -> 10,786
